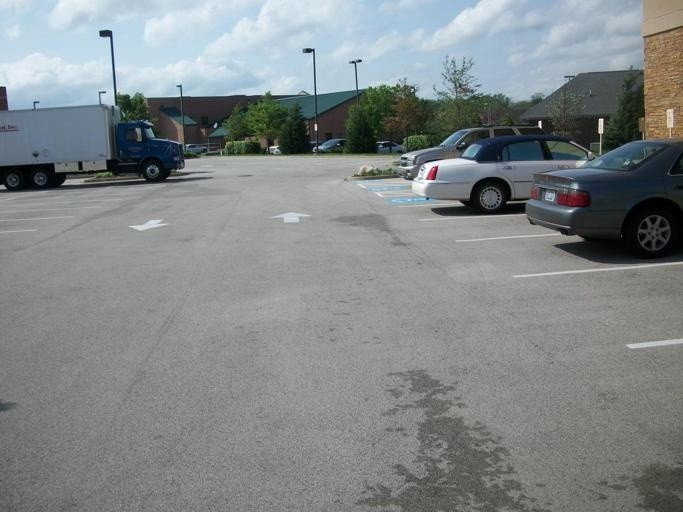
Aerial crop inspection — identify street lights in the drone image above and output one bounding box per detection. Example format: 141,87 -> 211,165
563,75 -> 575,82
32,101 -> 39,109
302,48 -> 320,156
98,90 -> 106,105
98,30 -> 118,106
348,59 -> 362,105
175,84 -> 185,145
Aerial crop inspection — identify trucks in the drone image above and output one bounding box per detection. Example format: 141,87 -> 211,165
0,103 -> 185,191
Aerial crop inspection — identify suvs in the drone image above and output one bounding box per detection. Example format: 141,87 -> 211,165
394,123 -> 548,181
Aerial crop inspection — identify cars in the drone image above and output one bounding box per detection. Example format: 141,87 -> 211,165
410,132 -> 598,217
264,137 -> 406,157
522,135 -> 683,260
182,143 -> 208,155
